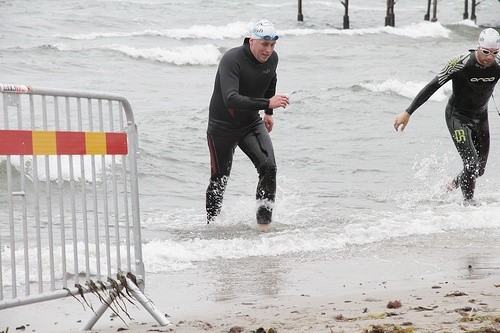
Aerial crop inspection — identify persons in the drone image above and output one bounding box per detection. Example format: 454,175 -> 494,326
205,19 -> 290,233
393,28 -> 500,207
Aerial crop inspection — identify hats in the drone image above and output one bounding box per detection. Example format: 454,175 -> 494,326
477,28 -> 500,49
251,19 -> 276,39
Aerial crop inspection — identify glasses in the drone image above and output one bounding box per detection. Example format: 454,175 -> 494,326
252,31 -> 279,42
479,47 -> 500,56
